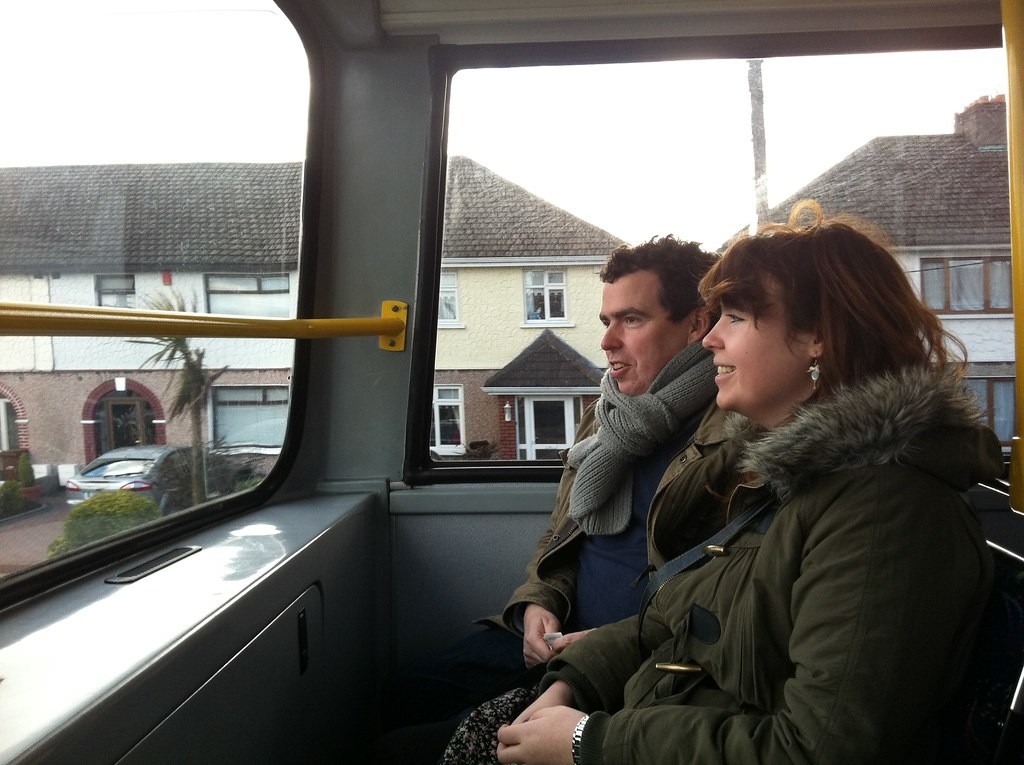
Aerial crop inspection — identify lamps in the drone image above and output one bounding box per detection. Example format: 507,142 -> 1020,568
504,400 -> 512,421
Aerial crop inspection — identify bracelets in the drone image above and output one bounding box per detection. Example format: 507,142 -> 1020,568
571,715 -> 589,765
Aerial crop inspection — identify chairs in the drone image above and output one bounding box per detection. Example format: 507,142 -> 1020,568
960,480 -> 1023,765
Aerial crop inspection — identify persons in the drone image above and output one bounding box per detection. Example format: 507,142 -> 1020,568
442,235 -> 743,717
441,198 -> 1005,765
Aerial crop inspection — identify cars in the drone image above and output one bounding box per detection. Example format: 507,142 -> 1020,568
65,444 -> 252,522
